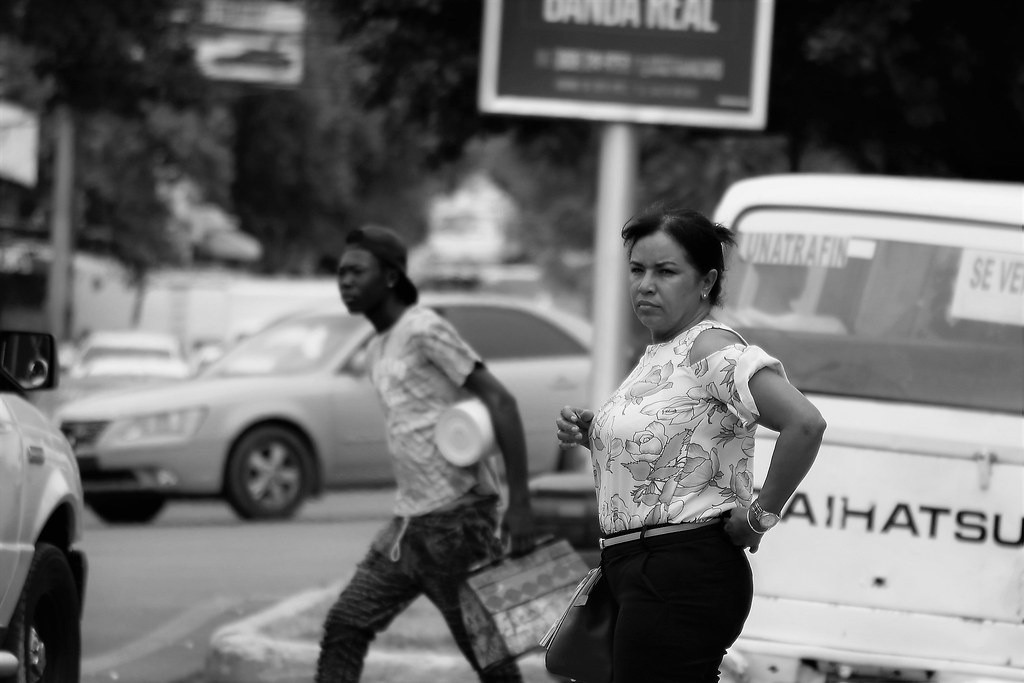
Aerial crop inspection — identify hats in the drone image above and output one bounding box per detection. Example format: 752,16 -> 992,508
345,225 -> 418,307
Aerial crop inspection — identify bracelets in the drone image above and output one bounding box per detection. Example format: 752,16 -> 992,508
746,510 -> 766,534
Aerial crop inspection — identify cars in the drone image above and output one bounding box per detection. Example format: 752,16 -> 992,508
49,282 -> 635,551
3,106 -> 311,682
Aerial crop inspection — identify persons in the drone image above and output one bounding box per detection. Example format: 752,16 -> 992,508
314,226 -> 536,683
545,206 -> 825,683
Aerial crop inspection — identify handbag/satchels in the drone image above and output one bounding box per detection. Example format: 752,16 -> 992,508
457,535 -> 592,670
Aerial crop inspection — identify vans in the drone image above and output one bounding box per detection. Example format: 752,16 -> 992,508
686,169 -> 1022,683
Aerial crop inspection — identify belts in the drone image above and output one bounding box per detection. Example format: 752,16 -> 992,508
599,518 -> 723,550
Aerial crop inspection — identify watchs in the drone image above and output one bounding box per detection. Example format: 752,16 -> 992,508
751,500 -> 779,529
